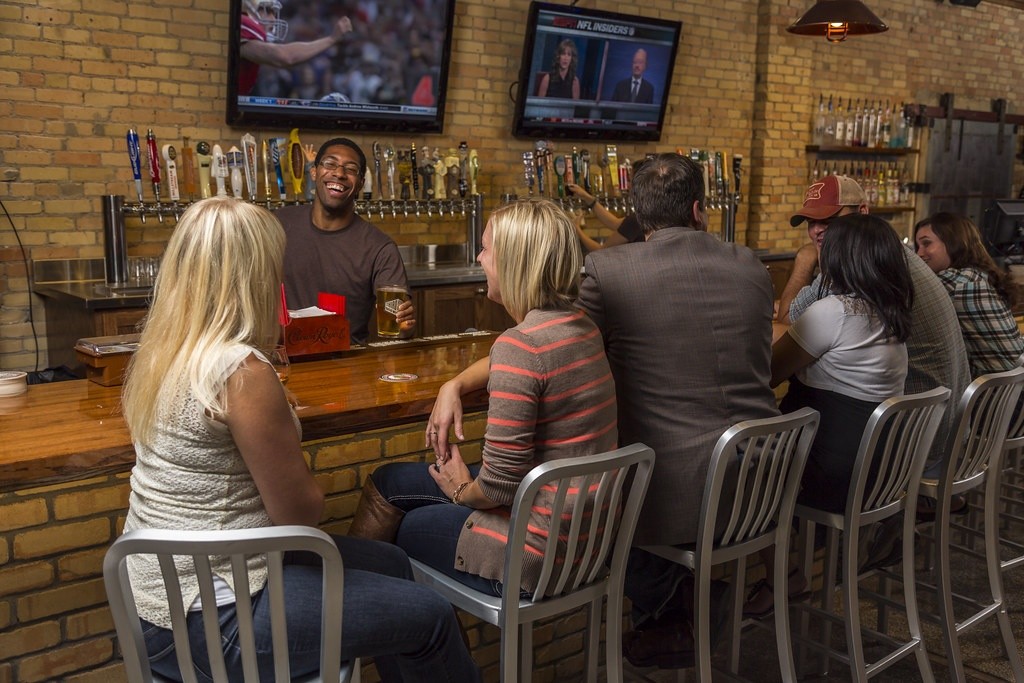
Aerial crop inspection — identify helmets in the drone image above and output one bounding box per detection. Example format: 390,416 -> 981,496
241,0 -> 288,43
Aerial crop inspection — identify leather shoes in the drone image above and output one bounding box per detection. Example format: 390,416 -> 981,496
683,576 -> 729,655
621,629 -> 697,670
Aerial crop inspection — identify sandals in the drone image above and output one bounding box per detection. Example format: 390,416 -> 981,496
742,566 -> 812,620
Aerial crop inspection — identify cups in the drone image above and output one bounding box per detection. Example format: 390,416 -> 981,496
560,195 -> 587,230
125,254 -> 164,279
256,345 -> 290,390
375,285 -> 409,338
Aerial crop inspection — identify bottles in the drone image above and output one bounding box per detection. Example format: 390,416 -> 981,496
807,93 -> 912,206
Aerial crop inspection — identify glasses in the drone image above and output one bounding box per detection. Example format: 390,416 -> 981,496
319,159 -> 363,177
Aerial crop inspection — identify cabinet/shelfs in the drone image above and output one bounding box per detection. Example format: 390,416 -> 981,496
797,140 -> 923,243
41,259 -> 794,370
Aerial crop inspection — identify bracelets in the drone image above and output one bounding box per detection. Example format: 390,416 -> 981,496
453,480 -> 473,505
588,197 -> 598,209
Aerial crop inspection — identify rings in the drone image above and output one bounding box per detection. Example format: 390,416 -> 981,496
430,431 -> 437,434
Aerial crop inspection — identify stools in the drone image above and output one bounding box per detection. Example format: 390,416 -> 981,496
879,366 -> 1024,683
964,353 -> 1024,556
406,444 -> 656,683
104,526 -> 362,683
625,409 -> 818,682
779,385 -> 951,683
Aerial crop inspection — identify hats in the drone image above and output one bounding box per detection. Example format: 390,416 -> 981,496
789,174 -> 868,227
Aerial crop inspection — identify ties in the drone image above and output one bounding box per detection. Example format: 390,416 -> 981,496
632,81 -> 639,102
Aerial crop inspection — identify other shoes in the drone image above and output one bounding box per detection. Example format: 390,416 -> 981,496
859,513 -> 921,572
916,494 -> 971,523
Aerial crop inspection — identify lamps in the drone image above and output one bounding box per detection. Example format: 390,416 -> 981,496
787,0 -> 887,43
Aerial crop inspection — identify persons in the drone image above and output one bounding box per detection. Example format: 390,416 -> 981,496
606,46 -> 655,124
563,152 -> 1024,671
275,137 -> 417,341
125,195 -> 481,683
239,0 -> 444,106
352,197 -> 618,595
539,38 -> 581,98
